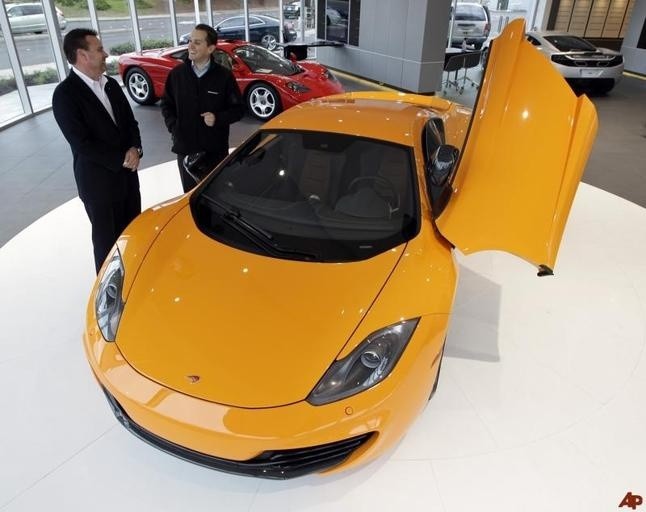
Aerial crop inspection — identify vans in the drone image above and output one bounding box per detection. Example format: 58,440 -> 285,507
445,3 -> 491,50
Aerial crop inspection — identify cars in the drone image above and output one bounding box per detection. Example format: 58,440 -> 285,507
179,15 -> 297,52
283,0 -> 300,17
3,4 -> 67,34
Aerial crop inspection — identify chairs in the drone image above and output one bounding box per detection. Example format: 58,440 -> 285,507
287,142 -> 406,212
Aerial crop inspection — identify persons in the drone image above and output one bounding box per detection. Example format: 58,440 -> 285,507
161,24 -> 244,193
50,28 -> 143,279
